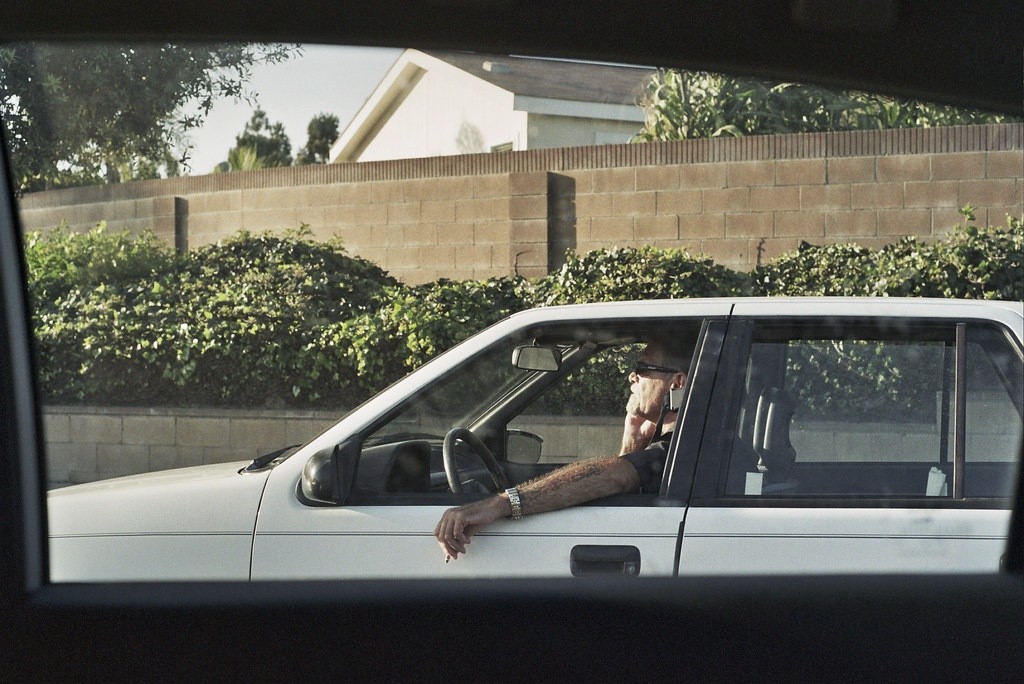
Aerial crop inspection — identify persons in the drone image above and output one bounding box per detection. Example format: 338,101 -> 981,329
434,331 -> 701,563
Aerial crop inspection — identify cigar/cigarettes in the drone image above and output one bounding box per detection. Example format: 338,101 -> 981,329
444,555 -> 451,563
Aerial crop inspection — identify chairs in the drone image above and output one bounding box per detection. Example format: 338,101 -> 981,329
747,384 -> 800,494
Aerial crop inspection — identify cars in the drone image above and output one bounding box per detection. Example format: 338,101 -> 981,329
42,294 -> 1023,583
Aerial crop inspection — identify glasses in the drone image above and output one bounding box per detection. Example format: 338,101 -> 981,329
633,361 -> 688,375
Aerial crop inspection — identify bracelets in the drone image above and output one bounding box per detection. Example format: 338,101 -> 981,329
505,488 -> 523,520
